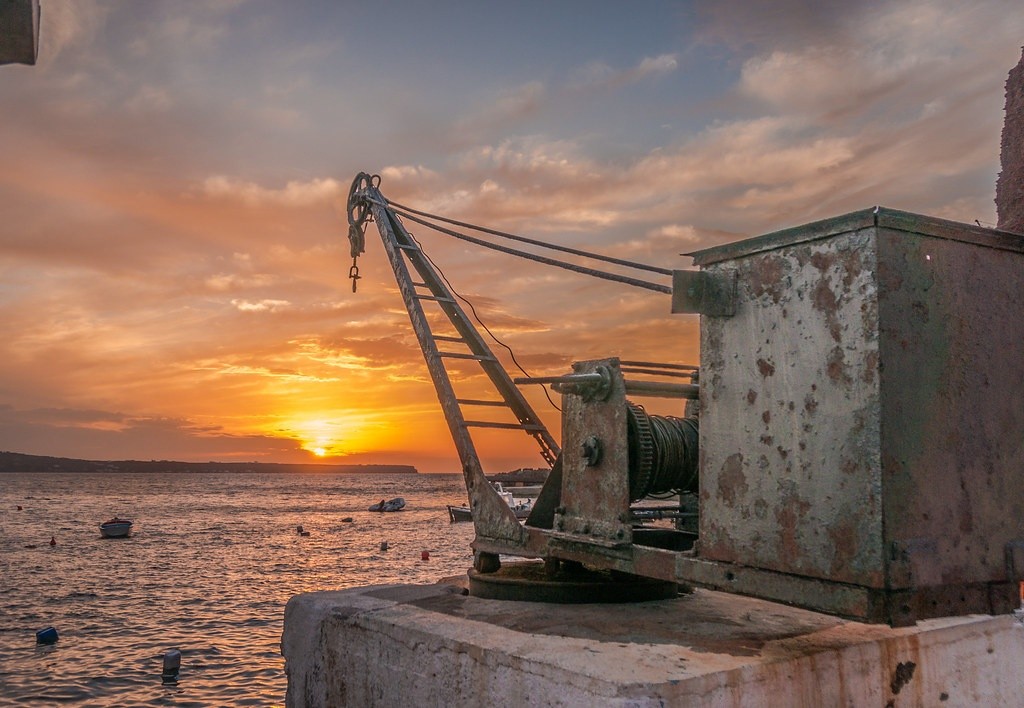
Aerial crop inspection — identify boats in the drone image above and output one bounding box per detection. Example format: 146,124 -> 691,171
369,498 -> 405,512
446,482 -> 534,523
100,520 -> 131,537
